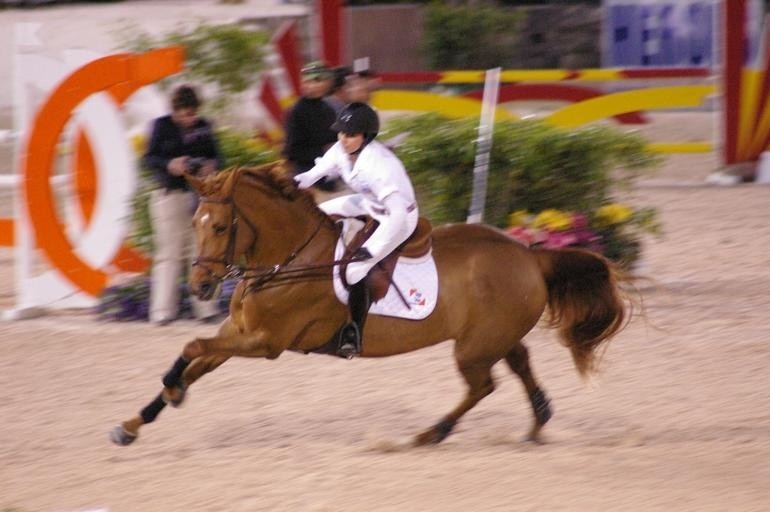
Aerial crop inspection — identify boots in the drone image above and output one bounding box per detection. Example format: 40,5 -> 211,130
336,273 -> 370,358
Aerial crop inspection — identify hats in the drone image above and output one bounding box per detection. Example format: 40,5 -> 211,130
301,60 -> 337,84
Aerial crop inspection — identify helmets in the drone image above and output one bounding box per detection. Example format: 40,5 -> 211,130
329,102 -> 379,142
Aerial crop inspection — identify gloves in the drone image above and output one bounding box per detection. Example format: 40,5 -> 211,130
347,248 -> 371,262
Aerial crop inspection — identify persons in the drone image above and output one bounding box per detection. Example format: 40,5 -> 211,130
285,60 -> 386,188
293,102 -> 421,360
144,83 -> 223,327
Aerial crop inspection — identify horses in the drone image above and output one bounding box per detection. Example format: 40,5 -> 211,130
110,163 -> 655,447
